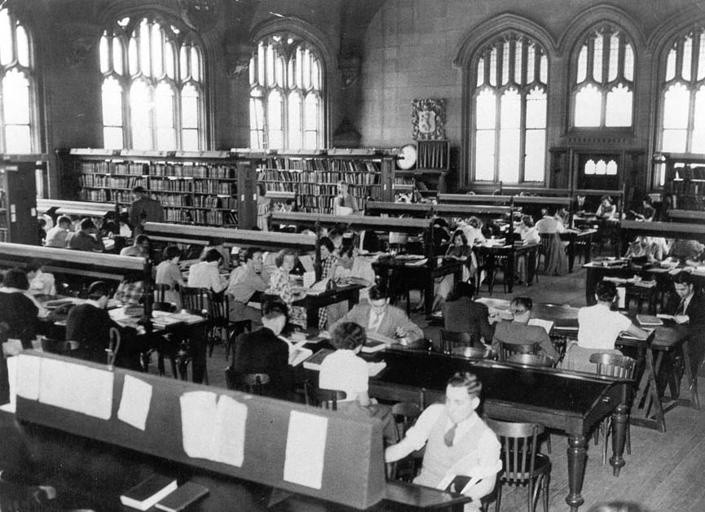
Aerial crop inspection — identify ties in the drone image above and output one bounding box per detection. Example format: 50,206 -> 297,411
676,299 -> 685,316
367,313 -> 380,331
444,424 -> 459,447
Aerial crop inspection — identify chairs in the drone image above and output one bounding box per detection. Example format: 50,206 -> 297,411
1,147 -> 704,512
